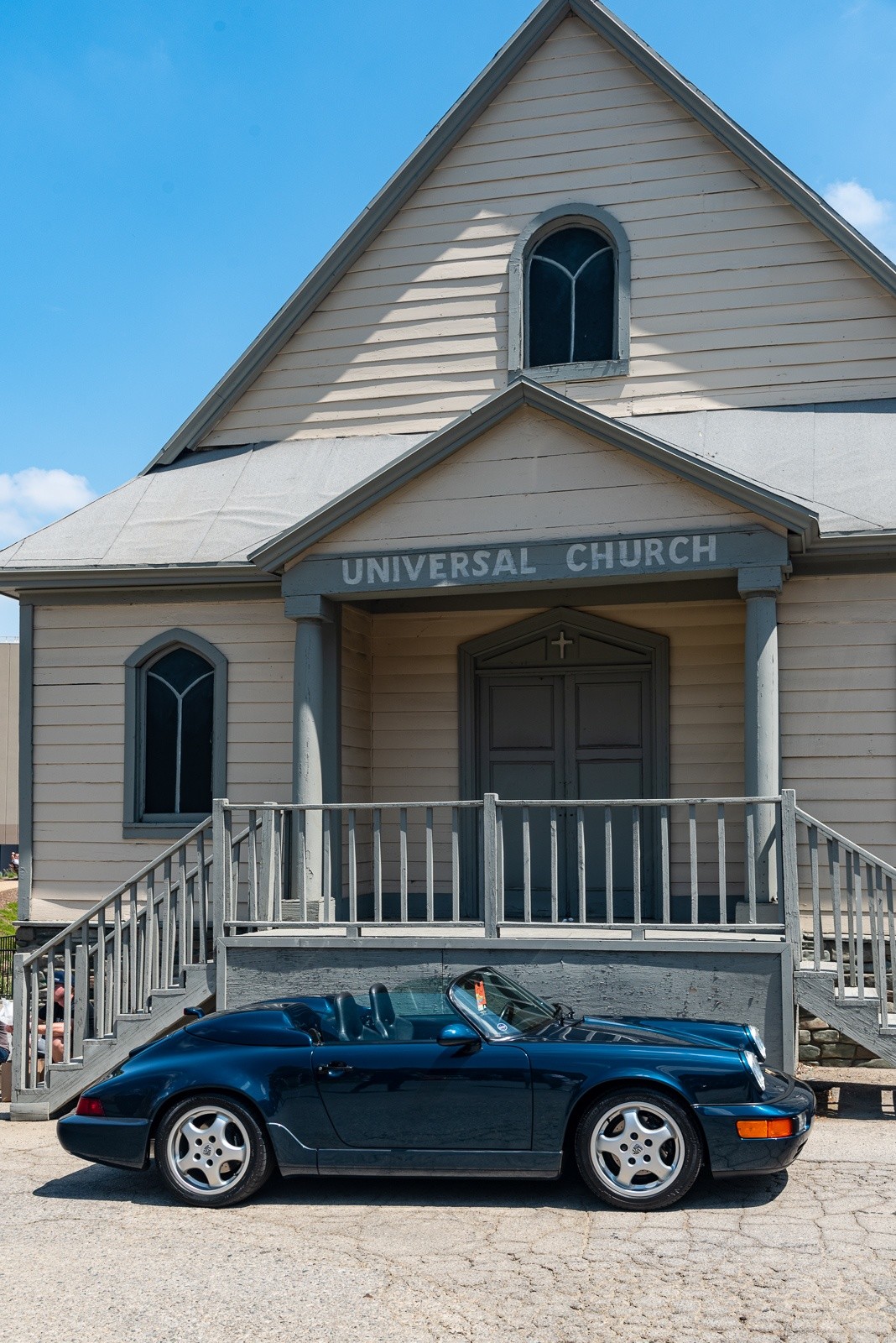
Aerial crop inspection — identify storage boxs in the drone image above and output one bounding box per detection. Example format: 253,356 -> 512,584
0,1058 -> 46,1102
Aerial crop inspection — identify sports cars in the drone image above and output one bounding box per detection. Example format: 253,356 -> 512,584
58,966 -> 816,1212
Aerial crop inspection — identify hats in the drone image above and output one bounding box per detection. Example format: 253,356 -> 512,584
46,971 -> 75,986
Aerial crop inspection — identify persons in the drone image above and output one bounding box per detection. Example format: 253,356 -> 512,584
0,998 -> 14,1102
11,853 -> 19,867
6,971 -> 94,1063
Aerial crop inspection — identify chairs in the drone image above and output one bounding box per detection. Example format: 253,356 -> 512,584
335,991 -> 391,1044
369,982 -> 438,1042
